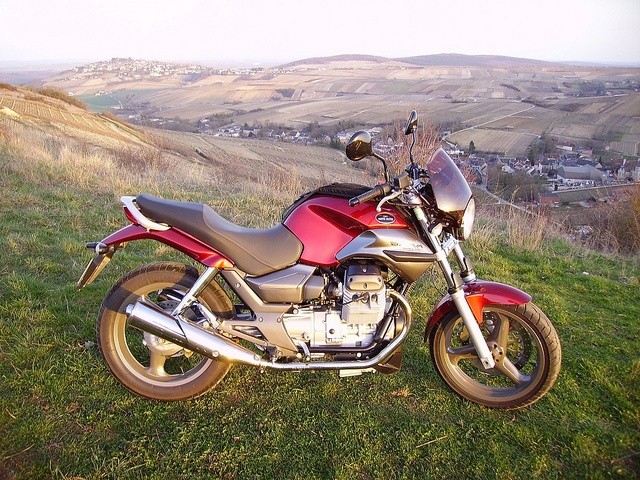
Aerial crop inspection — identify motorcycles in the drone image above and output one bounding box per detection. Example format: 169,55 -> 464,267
74,107 -> 564,411
